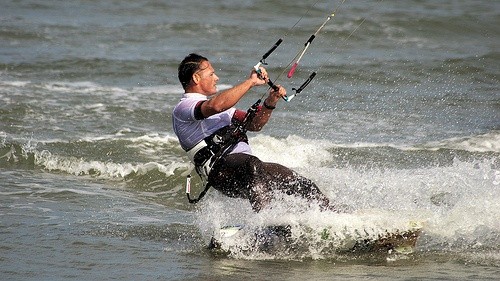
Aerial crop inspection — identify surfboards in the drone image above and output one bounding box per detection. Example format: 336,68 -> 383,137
209,224 -> 421,252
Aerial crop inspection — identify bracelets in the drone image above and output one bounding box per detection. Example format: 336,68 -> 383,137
263,98 -> 277,111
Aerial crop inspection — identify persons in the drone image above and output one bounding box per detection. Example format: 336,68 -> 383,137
171,52 -> 336,215
